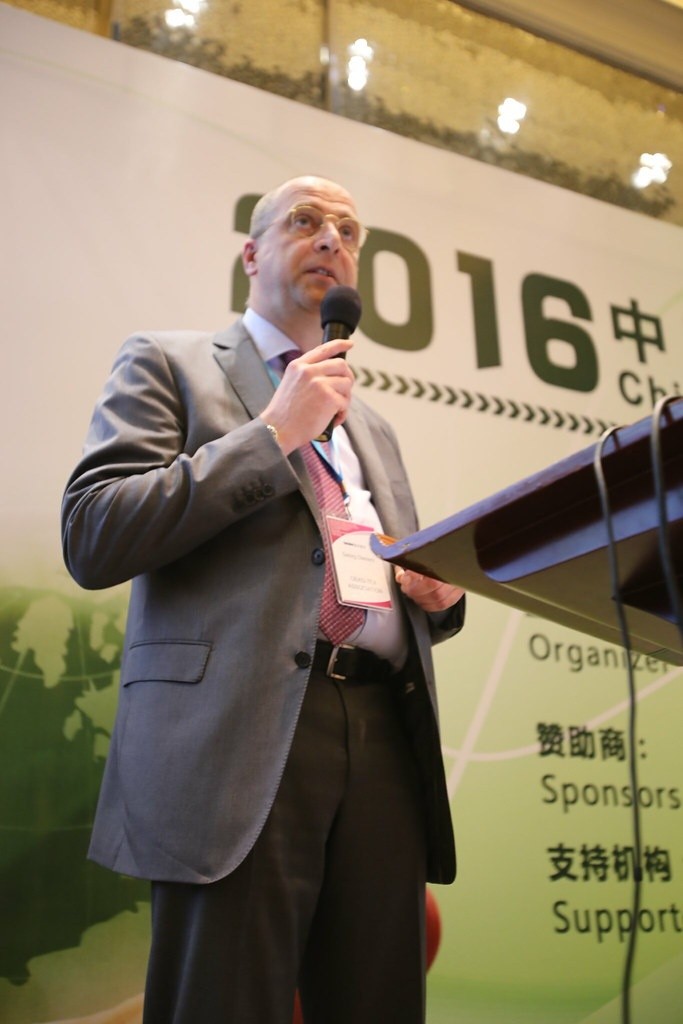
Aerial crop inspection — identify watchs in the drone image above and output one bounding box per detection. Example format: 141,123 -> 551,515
266,425 -> 282,448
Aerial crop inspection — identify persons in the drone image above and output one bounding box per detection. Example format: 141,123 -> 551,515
61,176 -> 468,1024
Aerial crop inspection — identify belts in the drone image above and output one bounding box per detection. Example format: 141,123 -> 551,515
309,639 -> 397,688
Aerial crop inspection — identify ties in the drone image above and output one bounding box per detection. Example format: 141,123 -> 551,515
280,349 -> 366,648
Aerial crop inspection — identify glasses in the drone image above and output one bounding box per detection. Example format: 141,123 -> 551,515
253,204 -> 370,253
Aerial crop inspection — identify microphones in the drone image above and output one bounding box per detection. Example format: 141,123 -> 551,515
315,285 -> 362,442
